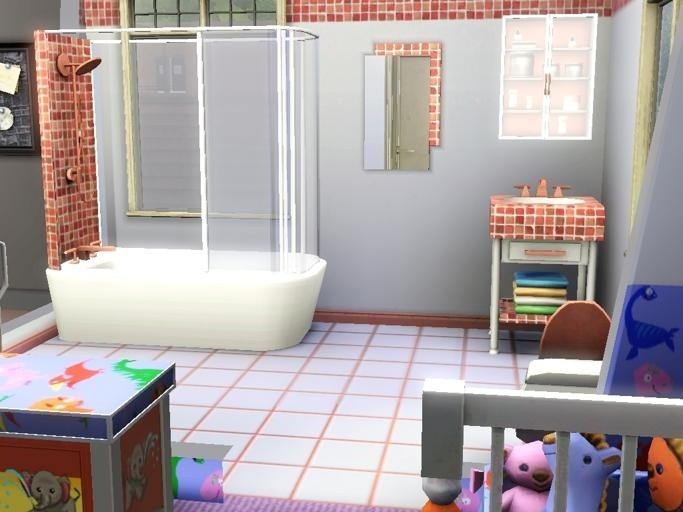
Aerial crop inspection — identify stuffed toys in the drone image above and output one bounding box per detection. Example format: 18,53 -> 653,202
501,431 -> 683,512
